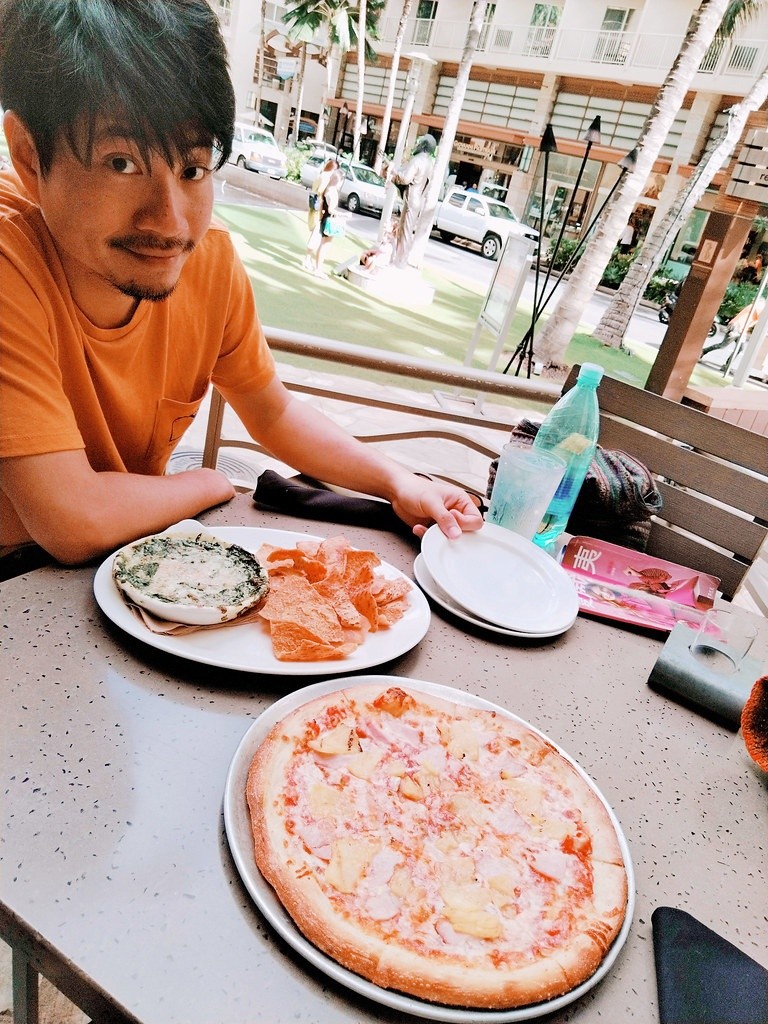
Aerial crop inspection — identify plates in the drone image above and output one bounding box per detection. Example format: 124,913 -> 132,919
93,527 -> 431,675
413,553 -> 575,637
225,676 -> 634,1022
112,520 -> 268,625
421,519 -> 579,633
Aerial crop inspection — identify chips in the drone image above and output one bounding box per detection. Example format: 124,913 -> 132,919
256,534 -> 415,661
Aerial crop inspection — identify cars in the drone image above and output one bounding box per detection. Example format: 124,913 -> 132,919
212,121 -> 287,181
299,148 -> 402,216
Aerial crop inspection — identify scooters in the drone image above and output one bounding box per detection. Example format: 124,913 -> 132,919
657,276 -> 722,338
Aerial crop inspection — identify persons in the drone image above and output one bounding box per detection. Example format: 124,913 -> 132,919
699,296 -> 765,376
0,0 -> 483,583
333,220 -> 398,279
313,170 -> 345,281
301,160 -> 339,272
382,134 -> 437,268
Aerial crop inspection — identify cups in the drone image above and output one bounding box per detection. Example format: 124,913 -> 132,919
486,443 -> 568,541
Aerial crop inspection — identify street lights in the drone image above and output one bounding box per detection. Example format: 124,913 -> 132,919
394,51 -> 436,172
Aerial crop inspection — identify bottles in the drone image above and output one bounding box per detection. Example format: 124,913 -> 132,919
532,363 -> 604,547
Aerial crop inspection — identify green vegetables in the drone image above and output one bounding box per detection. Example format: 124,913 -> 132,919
111,533 -> 266,605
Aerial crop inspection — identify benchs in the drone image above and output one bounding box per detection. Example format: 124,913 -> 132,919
681,386 -> 768,438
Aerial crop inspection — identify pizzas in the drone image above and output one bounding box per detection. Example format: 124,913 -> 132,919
247,684 -> 627,1008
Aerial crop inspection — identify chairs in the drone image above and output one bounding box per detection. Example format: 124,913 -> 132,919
557,364 -> 768,602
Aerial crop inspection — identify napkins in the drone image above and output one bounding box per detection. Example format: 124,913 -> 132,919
651,907 -> 768,1024
253,469 -> 411,536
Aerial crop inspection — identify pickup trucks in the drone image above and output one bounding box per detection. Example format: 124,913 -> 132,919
432,183 -> 540,264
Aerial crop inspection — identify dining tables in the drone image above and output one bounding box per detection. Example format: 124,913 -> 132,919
0,474 -> 768,1024
